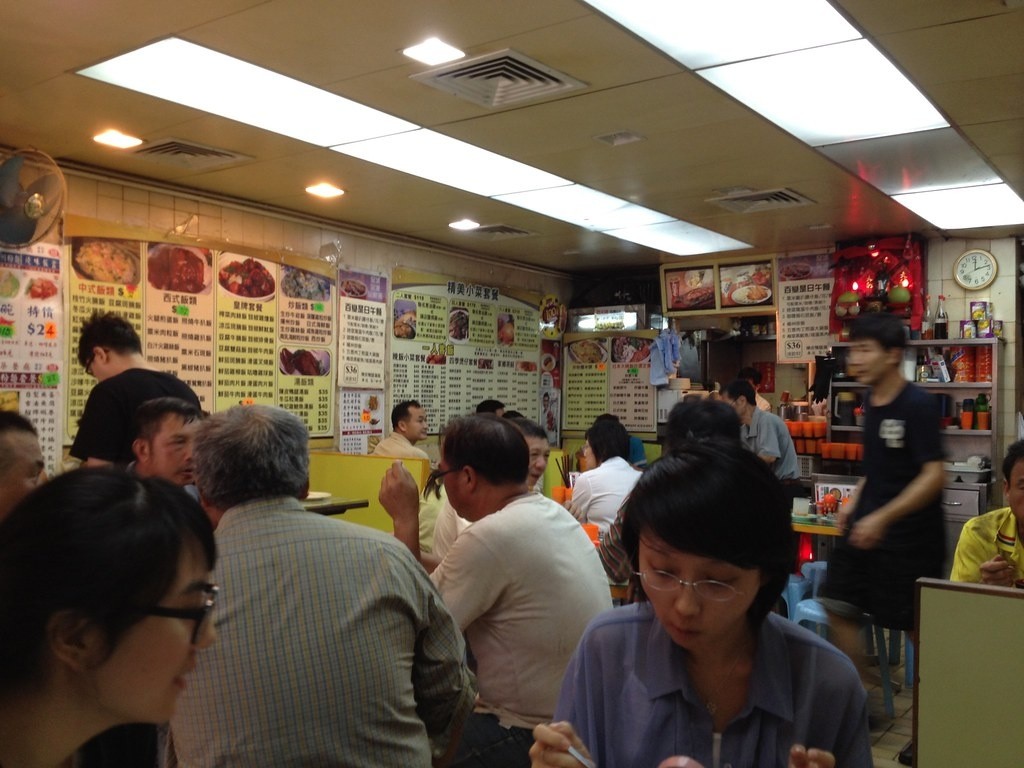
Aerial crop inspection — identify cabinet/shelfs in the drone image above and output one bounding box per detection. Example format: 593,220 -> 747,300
823,332 -> 1007,549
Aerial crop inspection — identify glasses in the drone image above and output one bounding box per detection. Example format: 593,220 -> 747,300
141,582 -> 220,645
85,348 -> 110,376
434,464 -> 462,486
629,547 -> 760,601
580,444 -> 589,453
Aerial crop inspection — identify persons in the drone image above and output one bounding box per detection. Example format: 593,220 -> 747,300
168,403 -> 477,768
663,400 -> 741,447
0,467 -> 218,768
68,311 -> 201,468
721,380 -> 799,479
873,263 -> 889,296
128,397 -> 199,503
367,400 -> 428,459
571,420 -> 643,540
0,412 -> 47,522
476,400 -> 507,417
711,369 -> 771,412
380,412 -> 613,768
503,410 -> 523,417
596,413 -> 649,471
834,313 -> 945,686
899,443 -> 1024,768
432,417 -> 583,561
528,442 -> 875,768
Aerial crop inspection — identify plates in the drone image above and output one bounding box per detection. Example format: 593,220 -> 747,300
690,383 -> 703,391
449,310 -> 469,344
731,286 -> 771,304
821,517 -> 837,526
306,491 -> 331,499
791,514 -> 817,522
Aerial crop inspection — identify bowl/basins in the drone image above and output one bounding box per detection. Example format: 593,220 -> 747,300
570,340 -> 607,363
279,348 -> 330,376
73,241 -> 367,302
669,379 -> 690,390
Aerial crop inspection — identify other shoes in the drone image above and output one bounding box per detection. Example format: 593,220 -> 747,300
868,714 -> 878,731
898,742 -> 912,766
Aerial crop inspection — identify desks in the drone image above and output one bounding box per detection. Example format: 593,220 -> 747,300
303,489 -> 369,519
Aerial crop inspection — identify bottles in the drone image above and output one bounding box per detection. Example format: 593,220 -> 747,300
742,317 -> 775,337
921,295 -> 948,340
790,402 -> 809,421
956,393 -> 991,429
808,495 -> 835,515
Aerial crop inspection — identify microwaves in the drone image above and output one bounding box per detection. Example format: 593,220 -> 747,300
658,391 -> 709,423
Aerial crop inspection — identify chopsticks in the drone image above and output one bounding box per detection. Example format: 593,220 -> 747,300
555,455 -> 573,488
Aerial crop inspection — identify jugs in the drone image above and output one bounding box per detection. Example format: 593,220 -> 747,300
835,392 -> 856,426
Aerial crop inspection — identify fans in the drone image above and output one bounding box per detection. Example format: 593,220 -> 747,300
0,148 -> 68,248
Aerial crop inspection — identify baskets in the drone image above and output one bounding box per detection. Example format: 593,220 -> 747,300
795,455 -> 821,478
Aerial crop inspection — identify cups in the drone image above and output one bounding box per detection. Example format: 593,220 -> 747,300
898,349 -> 917,381
552,487 -> 565,505
669,277 -> 679,299
784,421 -> 863,461
857,416 -> 865,426
793,498 -> 809,516
961,412 -> 973,430
565,488 -> 573,501
581,523 -> 598,542
977,412 -> 989,430
780,391 -> 789,404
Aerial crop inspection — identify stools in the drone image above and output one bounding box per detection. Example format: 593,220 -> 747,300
779,560 -> 914,720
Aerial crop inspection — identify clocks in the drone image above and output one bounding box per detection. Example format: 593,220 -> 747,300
954,249 -> 999,292
